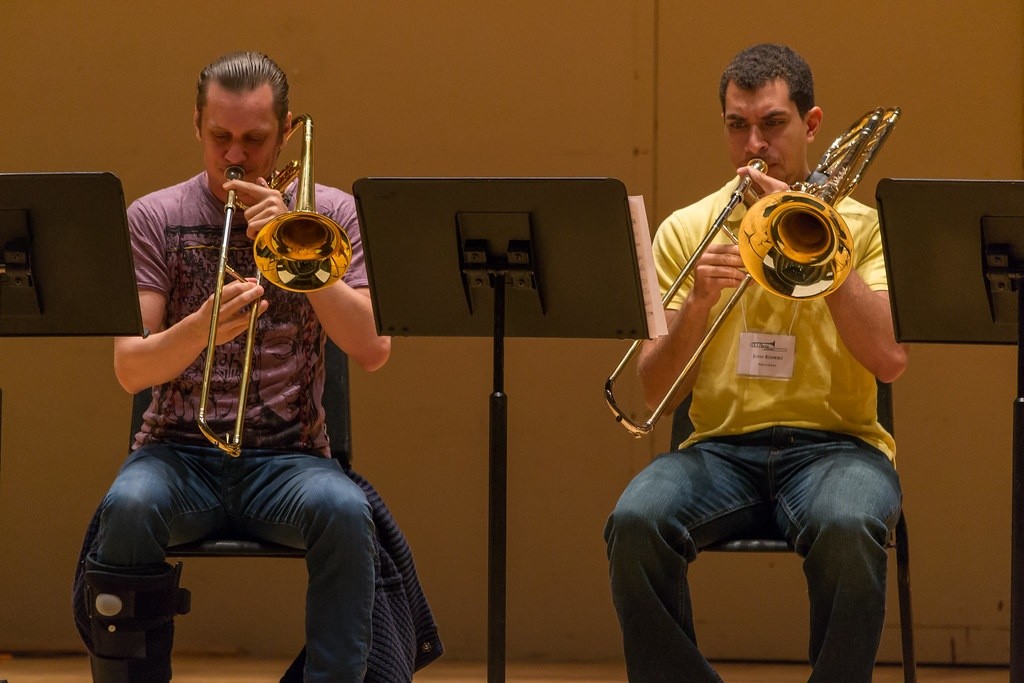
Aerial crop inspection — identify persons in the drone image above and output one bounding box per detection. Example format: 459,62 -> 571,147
81,51 -> 394,683
602,42 -> 910,683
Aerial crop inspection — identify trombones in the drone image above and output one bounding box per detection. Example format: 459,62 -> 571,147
602,103 -> 904,441
199,111 -> 355,465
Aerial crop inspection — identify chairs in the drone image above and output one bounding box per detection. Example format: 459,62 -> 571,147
128,336 -> 353,560
671,378 -> 917,683
